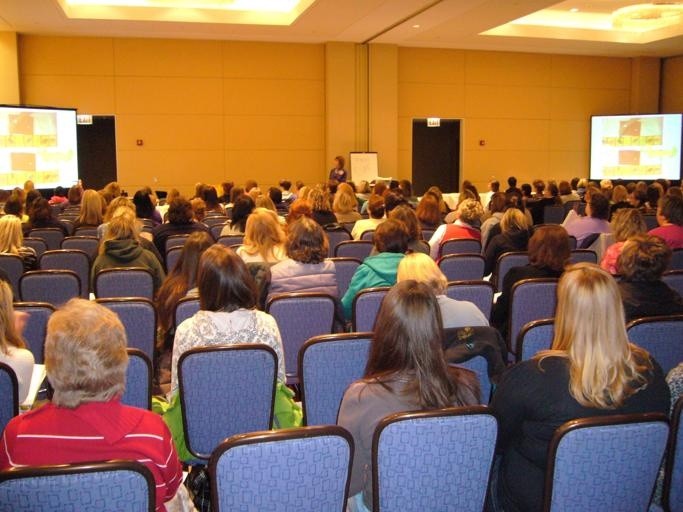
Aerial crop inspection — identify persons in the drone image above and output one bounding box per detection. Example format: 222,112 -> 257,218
577,179 -> 590,198
246,178 -> 259,193
255,195 -> 287,224
109,181 -> 122,196
630,190 -> 648,214
571,176 -> 581,197
389,178 -> 400,192
73,189 -> 104,232
68,179 -> 85,206
637,181 -> 651,202
441,189 -> 486,226
427,198 -> 483,263
645,194 -> 682,250
614,234 -> 682,326
558,180 -> 581,205
482,207 -> 531,279
267,186 -> 290,211
396,178 -> 419,206
154,231 -> 218,350
395,250 -> 490,328
289,185 -> 313,209
23,197 -> 70,237
295,180 -> 305,189
577,184 -> 603,218
145,186 -> 157,206
90,216 -> 168,350
486,180 -> 503,206
649,361 -> 683,505
599,178 -> 615,201
532,180 -> 562,225
331,183 -> 362,223
11,185 -> 24,200
265,217 -> 339,311
158,188 -> 185,223
99,190 -> 114,208
430,186 -> 453,213
133,190 -> 163,228
463,178 -> 482,204
219,195 -> 258,237
521,181 -> 534,209
96,195 -> 149,242
225,186 -> 245,208
1,214 -> 38,274
235,207 -> 290,263
1,277 -> 35,419
417,192 -> 443,231
277,178 -> 299,204
646,182 -> 665,209
202,185 -> 227,213
315,181 -> 333,197
153,198 -> 218,258
219,181 -> 234,200
669,186 -> 681,197
486,260 -> 670,510
347,183 -> 367,207
479,193 -> 506,256
1,195 -> 33,230
308,188 -> 338,225
189,179 -> 204,200
608,185 -> 637,223
386,191 -> 412,213
589,181 -> 597,188
368,205 -> 432,256
343,218 -> 410,333
626,181 -> 641,207
657,178 -> 672,192
491,223 -> 575,318
599,206 -> 649,274
506,190 -> 535,227
247,186 -> 262,202
565,193 -> 615,250
326,156 -> 350,195
503,176 -> 522,203
25,189 -> 41,214
23,180 -> 34,197
192,198 -> 210,223
1,298 -> 193,512
334,280 -> 483,510
47,185 -> 70,209
350,194 -> 386,240
285,198 -> 315,228
168,243 -> 288,403
360,180 -> 389,214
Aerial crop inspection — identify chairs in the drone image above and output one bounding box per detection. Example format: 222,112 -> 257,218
371,405 -> 499,510
11,301 -> 56,403
260,295 -> 332,386
351,286 -> 390,335
162,199 -> 484,295
623,310 -> 683,377
662,395 -> 683,512
92,298 -> 155,374
177,344 -> 279,457
1,362 -> 19,439
541,411 -> 671,512
0,198 -> 155,305
516,320 -> 562,364
1,458 -> 156,511
203,424 -> 356,512
172,297 -> 206,333
494,210 -> 683,299
442,279 -> 494,323
505,275 -> 560,362
297,333 -> 380,427
115,347 -> 152,414
